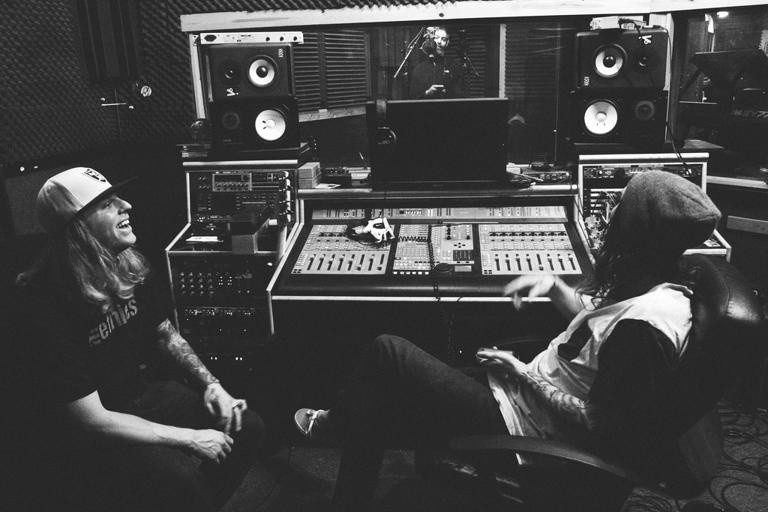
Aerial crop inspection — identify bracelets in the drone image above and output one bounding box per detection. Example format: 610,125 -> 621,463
552,274 -> 561,301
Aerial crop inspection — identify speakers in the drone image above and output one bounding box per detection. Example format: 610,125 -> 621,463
576,31 -> 668,87
572,88 -> 668,143
209,95 -> 299,147
207,45 -> 292,95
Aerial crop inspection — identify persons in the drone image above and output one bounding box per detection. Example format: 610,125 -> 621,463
295,170 -> 722,512
0,164 -> 265,512
408,28 -> 470,98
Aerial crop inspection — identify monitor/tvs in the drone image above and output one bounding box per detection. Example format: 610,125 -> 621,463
367,98 -> 509,189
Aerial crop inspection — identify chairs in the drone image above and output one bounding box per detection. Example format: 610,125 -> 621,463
417,254 -> 765,511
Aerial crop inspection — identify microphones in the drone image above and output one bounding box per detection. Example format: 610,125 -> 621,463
423,39 -> 436,61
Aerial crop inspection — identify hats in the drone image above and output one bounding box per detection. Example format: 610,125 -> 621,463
35,166 -> 137,232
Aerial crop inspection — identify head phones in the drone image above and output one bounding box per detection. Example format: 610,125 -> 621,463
345,218 -> 390,242
372,99 -> 398,149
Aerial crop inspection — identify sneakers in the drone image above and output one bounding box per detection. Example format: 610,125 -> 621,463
294,406 -> 330,438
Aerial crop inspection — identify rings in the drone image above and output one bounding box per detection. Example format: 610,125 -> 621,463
233,401 -> 242,411
